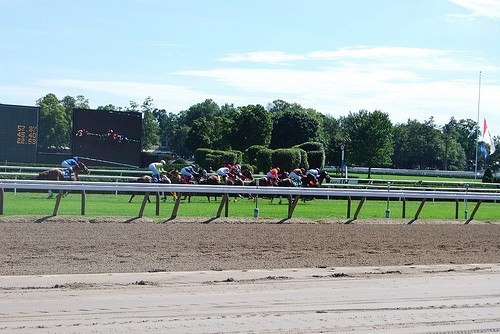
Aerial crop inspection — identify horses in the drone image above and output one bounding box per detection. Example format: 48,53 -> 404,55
128,167 -> 332,204
27,160 -> 91,199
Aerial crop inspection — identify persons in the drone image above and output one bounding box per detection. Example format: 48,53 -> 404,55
306,168 -> 320,181
181,164 -> 199,179
217,164 -> 242,180
149,160 -> 167,176
290,168 -> 307,185
61,157 -> 80,173
267,167 -> 280,186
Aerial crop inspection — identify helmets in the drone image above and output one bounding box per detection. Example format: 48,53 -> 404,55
161,160 -> 166,164
192,164 -> 195,169
227,165 -> 231,168
316,168 -> 320,173
277,168 -> 280,171
301,168 -> 304,170
73,157 -> 78,161
